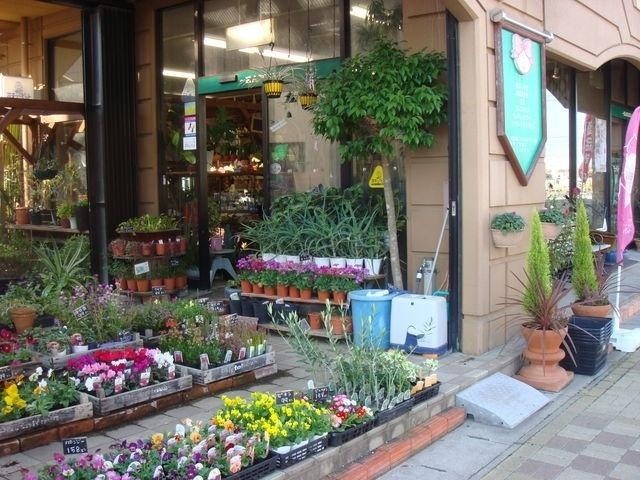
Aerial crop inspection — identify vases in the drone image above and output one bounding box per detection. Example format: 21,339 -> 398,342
561,317 -> 613,376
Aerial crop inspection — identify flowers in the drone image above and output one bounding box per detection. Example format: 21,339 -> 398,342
0,328 -> 375,480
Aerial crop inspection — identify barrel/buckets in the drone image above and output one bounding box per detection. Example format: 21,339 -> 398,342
345,288 -> 398,352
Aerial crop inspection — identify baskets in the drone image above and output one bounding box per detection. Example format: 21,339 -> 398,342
223,378 -> 441,480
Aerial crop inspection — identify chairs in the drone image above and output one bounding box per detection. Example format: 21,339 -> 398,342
590,232 -> 611,275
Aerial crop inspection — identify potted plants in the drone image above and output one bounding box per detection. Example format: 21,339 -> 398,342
491,205 -> 596,394
568,198 -> 640,353
490,210 -> 525,249
539,204 -> 566,242
250,42 -> 302,98
0,116 -> 407,329
295,57 -> 323,110
